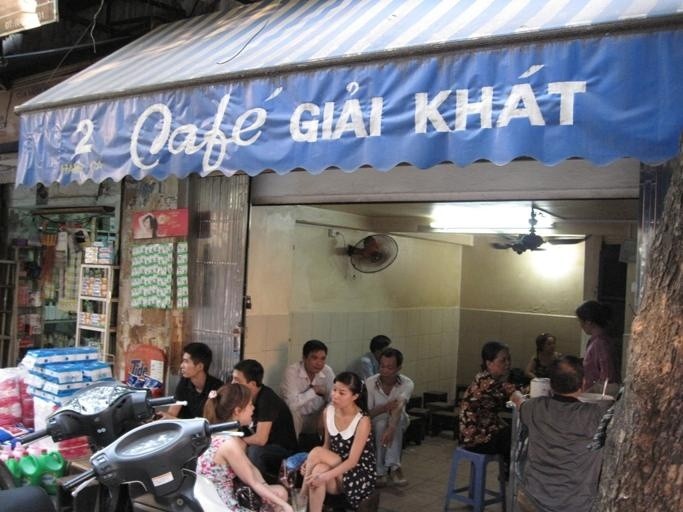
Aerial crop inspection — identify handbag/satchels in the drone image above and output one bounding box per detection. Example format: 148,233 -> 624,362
233,480 -> 262,509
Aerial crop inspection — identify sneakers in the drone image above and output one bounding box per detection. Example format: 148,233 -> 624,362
391,467 -> 407,486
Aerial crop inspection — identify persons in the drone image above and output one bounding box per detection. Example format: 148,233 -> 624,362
516,355 -> 615,512
151,336 -> 298,512
525,333 -> 563,379
279,336 -> 414,512
138,213 -> 158,238
575,300 -> 620,393
457,342 -> 530,480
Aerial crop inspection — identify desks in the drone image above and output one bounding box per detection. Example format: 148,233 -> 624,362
70,458 -> 101,512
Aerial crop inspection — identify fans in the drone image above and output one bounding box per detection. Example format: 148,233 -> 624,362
490,205 -> 591,255
347,233 -> 399,274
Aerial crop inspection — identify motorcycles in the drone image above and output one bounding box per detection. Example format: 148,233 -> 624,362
0,380 -> 188,512
0,460 -> 14,490
63,417 -> 244,512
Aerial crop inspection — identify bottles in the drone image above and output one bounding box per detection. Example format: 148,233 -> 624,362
0,441 -> 42,463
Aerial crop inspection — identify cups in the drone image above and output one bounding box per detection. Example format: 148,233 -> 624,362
292,494 -> 309,512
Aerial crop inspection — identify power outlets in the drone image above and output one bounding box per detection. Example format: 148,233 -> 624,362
328,228 -> 340,238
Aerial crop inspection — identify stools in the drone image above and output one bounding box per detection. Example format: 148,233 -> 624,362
324,489 -> 380,511
402,383 -> 471,448
443,444 -> 508,512
282,453 -> 308,476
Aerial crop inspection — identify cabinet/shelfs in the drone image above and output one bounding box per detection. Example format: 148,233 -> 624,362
10,244 -> 48,364
73,263 -> 120,364
40,225 -> 118,324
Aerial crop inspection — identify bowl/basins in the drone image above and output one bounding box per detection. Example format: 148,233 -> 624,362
579,392 -> 614,408
